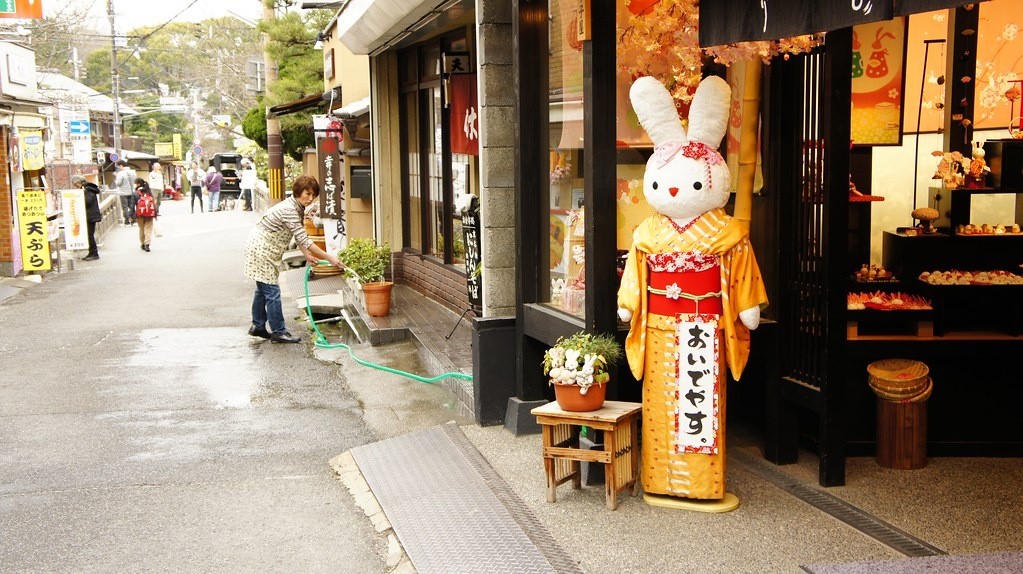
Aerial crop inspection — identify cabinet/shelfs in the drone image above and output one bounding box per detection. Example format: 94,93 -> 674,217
846,140 -> 1023,458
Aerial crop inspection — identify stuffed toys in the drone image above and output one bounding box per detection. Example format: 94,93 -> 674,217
620,75 -> 762,501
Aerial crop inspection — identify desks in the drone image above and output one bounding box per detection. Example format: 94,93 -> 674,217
531,400 -> 642,510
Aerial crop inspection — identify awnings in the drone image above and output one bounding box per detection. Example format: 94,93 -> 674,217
268,93 -> 331,121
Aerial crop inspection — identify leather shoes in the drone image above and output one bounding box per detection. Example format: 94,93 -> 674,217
271,332 -> 301,344
248,325 -> 271,339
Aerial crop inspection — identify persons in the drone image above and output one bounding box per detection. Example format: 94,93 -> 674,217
150,162 -> 164,216
206,164 -> 224,212
239,157 -> 256,210
129,179 -> 157,252
187,160 -> 205,213
115,159 -> 137,225
248,176 -> 346,344
72,175 -> 102,260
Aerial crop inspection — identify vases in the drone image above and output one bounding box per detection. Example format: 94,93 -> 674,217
362,281 -> 393,317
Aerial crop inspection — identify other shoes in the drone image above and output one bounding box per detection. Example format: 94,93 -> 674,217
141,244 -> 150,252
208,209 -> 221,212
124,219 -> 136,224
244,208 -> 252,211
157,214 -> 161,216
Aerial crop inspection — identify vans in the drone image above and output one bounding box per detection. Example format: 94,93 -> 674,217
209,154 -> 243,200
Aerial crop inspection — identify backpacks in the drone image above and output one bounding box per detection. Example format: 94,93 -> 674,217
135,190 -> 156,217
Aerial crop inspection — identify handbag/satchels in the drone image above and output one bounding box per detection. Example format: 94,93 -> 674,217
203,187 -> 209,195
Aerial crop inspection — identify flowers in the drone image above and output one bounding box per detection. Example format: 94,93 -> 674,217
681,140 -> 724,164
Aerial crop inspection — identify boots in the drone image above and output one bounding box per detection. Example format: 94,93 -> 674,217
82,250 -> 99,260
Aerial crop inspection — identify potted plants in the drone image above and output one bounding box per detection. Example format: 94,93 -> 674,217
340,236 -> 391,310
541,330 -> 625,412
